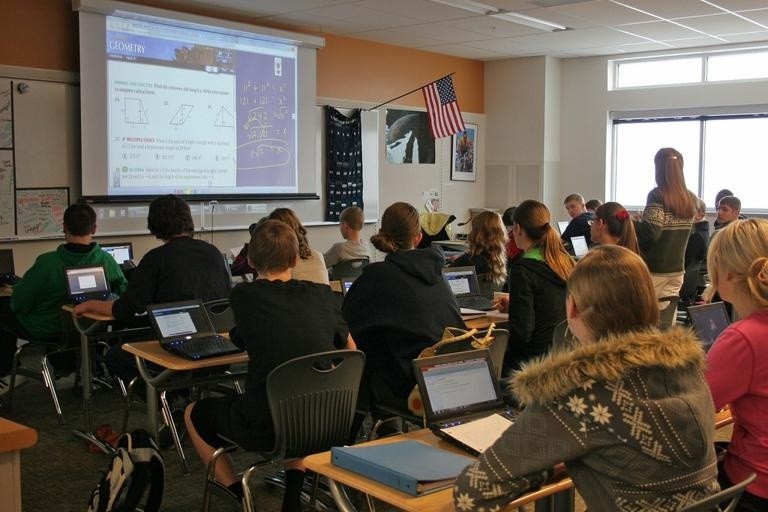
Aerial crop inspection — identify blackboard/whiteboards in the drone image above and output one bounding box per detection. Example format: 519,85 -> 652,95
0,63 -> 381,244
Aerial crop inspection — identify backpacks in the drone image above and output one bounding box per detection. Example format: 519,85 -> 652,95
87,428 -> 164,512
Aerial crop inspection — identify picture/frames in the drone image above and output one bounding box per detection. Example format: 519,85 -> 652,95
450,122 -> 478,182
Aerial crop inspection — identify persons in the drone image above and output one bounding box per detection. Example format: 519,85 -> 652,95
632,149 -> 702,324
0,199 -> 128,396
346,193 -> 768,512
228,206 -> 371,286
73,192 -> 232,449
184,220 -> 358,510
457,131 -> 473,173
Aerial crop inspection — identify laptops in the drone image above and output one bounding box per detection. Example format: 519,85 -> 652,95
686,301 -> 730,346
64,267 -> 117,304
570,235 -> 589,256
340,277 -> 355,293
557,221 -> 569,234
99,242 -> 133,266
144,298 -> 240,361
0,248 -> 17,285
411,348 -> 523,456
441,266 -> 495,312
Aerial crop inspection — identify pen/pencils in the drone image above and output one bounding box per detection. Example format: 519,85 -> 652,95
492,301 -> 503,308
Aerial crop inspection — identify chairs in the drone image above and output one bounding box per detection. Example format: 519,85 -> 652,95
202,297 -> 237,334
203,349 -> 367,511
680,474 -> 757,511
328,258 -> 369,284
367,327 -> 512,442
416,213 -> 457,248
659,295 -> 680,329
549,316 -> 572,345
7,284 -> 131,416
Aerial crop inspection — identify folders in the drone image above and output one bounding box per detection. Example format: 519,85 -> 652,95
331,441 -> 476,497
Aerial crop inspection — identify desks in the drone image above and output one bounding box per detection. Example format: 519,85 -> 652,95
432,239 -> 469,256
121,288 -> 510,455
301,405 -> 734,512
63,279 -> 342,456
0,418 -> 38,512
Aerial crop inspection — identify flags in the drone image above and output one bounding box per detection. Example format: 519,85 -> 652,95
421,77 -> 466,140
